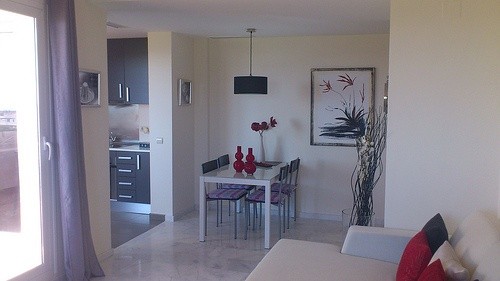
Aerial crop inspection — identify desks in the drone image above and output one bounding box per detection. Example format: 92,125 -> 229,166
198,161 -> 291,250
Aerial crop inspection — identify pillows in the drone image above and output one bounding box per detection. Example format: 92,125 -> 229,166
424,213 -> 449,254
427,240 -> 471,281
418,257 -> 447,281
395,229 -> 432,281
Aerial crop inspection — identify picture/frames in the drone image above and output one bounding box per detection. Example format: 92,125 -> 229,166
78,67 -> 102,108
177,78 -> 193,106
308,66 -> 376,148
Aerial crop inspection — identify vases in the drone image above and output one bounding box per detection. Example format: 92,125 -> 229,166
257,136 -> 266,162
233,145 -> 244,173
243,147 -> 257,176
340,208 -> 376,244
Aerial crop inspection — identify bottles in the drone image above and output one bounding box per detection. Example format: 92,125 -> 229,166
233,145 -> 244,172
244,148 -> 256,175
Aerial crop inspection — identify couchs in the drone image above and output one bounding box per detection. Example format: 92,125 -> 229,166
244,208 -> 500,281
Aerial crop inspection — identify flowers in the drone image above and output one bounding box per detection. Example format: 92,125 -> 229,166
251,115 -> 278,136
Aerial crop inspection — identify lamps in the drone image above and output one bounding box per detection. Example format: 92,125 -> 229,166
233,27 -> 267,96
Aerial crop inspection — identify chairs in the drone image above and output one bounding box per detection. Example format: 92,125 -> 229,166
243,164 -> 291,241
201,159 -> 251,241
217,154 -> 258,225
256,157 -> 302,229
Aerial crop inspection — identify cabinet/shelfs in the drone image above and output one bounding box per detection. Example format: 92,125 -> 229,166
108,151 -> 150,215
107,37 -> 149,105
